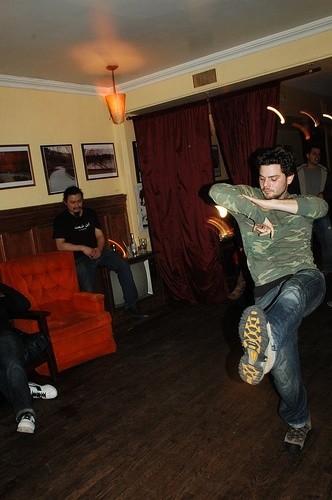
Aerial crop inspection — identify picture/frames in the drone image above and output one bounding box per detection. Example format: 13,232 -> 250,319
0,144 -> 36,189
211,144 -> 221,177
40,144 -> 79,195
132,140 -> 142,183
81,142 -> 119,180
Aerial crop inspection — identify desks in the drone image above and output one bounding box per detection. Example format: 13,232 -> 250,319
97,250 -> 160,327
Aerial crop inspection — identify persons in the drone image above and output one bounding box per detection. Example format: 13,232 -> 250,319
0,282 -> 57,434
208,146 -> 329,451
286,143 -> 332,308
52,186 -> 150,318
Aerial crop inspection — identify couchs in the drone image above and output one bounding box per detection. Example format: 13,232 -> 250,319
0,251 -> 117,375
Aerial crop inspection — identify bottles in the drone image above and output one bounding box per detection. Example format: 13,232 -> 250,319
130,233 -> 137,256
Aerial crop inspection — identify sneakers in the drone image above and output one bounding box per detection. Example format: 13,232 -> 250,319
28,382 -> 58,398
237,305 -> 276,385
17,415 -> 36,433
284,419 -> 313,450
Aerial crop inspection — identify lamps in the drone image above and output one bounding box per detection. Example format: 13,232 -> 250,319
105,64 -> 126,124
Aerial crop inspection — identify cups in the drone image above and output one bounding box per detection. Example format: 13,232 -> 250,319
138,237 -> 146,253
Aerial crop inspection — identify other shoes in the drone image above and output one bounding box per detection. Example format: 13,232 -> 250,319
127,306 -> 151,318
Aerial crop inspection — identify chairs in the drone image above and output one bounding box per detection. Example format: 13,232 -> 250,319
7,309 -> 61,385
